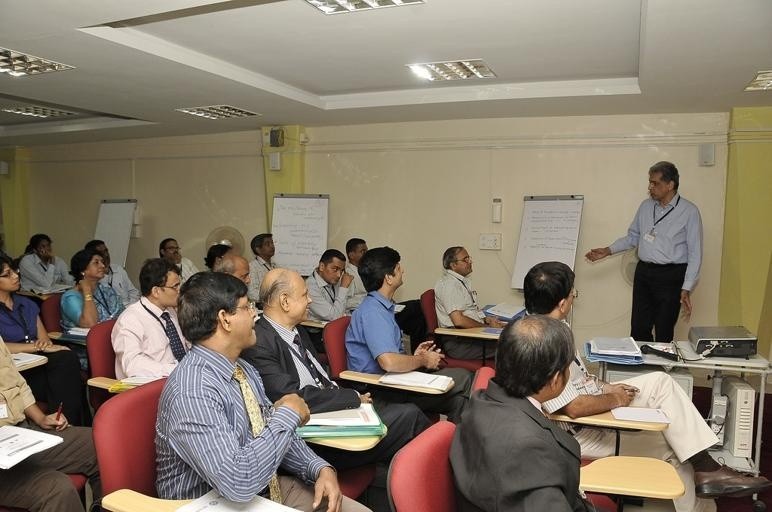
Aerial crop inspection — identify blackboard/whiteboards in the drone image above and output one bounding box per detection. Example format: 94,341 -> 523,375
511,195 -> 584,293
94,199 -> 137,268
271,194 -> 330,277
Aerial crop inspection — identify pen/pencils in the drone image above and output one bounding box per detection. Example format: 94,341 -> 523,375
53,402 -> 64,429
441,358 -> 449,365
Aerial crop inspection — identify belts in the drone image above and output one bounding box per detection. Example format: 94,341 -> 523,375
567,424 -> 583,437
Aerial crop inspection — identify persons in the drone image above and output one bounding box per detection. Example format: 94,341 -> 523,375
585,161 -> 703,343
435,246 -> 500,358
239,269 -> 435,481
1,252 -> 109,512
305,237 -> 369,323
522,261 -> 772,512
17,233 -> 281,379
448,317 -> 608,512
155,272 -> 374,512
343,246 -> 474,427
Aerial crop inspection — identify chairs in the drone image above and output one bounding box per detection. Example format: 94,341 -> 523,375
0,290 -> 686,511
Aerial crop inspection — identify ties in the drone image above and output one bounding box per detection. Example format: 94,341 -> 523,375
293,335 -> 338,390
161,312 -> 186,362
233,365 -> 282,503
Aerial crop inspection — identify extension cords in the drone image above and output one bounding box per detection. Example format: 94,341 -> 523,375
708,398 -> 726,446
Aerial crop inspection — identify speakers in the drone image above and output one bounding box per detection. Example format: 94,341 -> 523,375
269,151 -> 281,170
697,143 -> 716,166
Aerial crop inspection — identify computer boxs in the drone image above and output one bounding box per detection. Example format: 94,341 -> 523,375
722,375 -> 755,459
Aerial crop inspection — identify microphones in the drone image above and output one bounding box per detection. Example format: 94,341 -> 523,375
640,343 -> 678,361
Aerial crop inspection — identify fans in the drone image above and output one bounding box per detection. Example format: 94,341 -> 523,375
204,225 -> 246,259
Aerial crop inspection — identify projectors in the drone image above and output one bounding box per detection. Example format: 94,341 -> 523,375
690,325 -> 756,358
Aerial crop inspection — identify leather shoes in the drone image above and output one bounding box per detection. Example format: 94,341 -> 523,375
694,465 -> 772,498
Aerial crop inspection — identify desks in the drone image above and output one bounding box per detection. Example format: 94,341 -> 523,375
599,334 -> 772,478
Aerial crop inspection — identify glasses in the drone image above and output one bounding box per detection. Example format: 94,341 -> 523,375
455,256 -> 472,263
159,283 -> 179,290
0,268 -> 20,277
235,302 -> 254,310
565,288 -> 578,299
